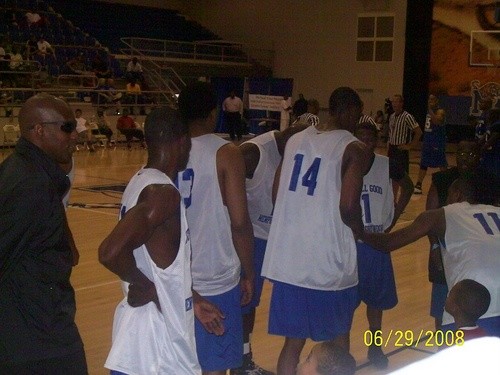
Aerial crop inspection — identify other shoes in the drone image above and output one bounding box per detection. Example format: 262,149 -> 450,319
230,360 -> 274,375
414,187 -> 423,194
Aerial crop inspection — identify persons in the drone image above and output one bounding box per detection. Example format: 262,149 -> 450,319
173,86 -> 254,375
0,93 -> 89,375
413,94 -> 448,194
222,88 -> 244,141
388,95 -> 422,207
0,6 -> 212,208
99,108 -> 226,374
237,87 -> 500,375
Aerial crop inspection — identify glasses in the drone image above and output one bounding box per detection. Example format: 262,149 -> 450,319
30,120 -> 77,132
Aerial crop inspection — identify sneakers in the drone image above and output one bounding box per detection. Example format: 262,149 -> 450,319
367,345 -> 389,368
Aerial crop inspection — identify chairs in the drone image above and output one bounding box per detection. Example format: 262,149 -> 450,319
2,124 -> 20,153
117,127 -> 127,148
87,121 -> 109,152
